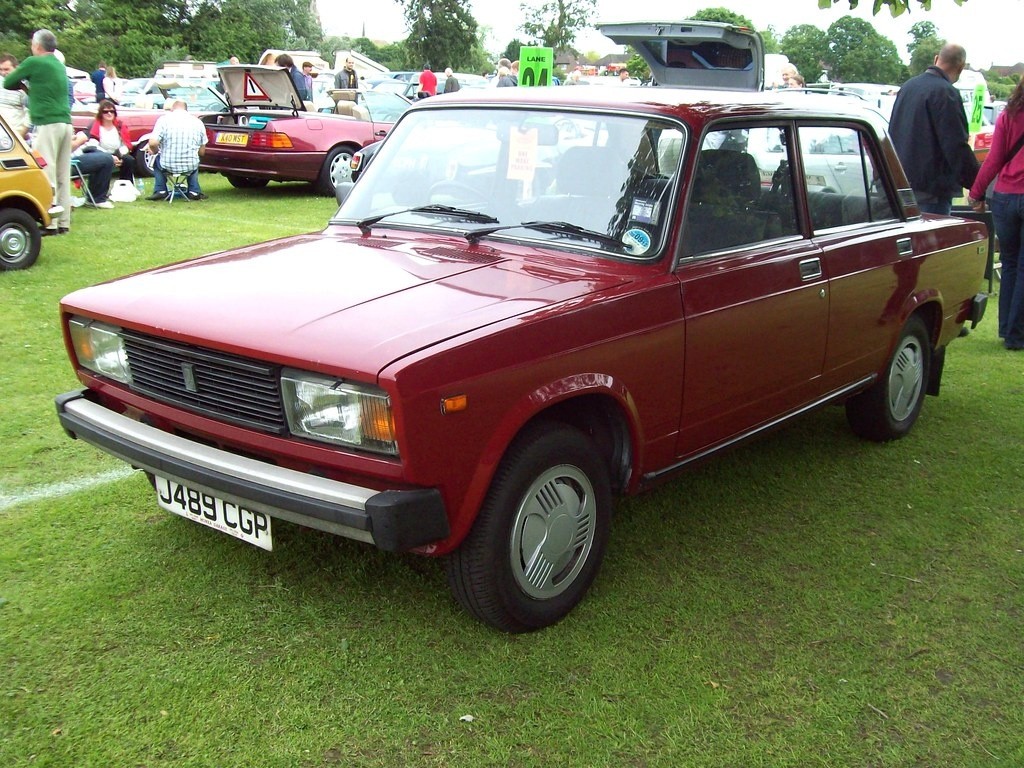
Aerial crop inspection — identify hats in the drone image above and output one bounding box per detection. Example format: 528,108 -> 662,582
303,62 -> 314,67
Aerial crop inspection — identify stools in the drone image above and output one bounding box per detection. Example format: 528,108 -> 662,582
159,169 -> 196,203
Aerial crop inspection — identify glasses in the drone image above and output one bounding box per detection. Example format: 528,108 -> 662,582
101,109 -> 115,114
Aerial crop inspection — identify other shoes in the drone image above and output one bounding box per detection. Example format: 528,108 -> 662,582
58,227 -> 68,234
38,226 -> 57,235
145,191 -> 170,201
1004,336 -> 1024,349
187,190 -> 208,201
957,328 -> 968,338
89,199 -> 114,208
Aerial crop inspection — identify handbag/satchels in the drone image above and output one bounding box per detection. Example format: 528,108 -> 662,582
109,180 -> 141,203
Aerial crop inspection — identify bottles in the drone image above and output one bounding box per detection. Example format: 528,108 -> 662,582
137,178 -> 145,195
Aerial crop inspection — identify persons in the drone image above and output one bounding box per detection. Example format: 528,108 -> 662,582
564,66 -> 581,85
228,54 -> 238,65
3,30 -> 72,237
498,58 -> 519,87
781,64 -> 796,88
611,69 -> 628,86
789,75 -> 805,88
103,66 -> 122,104
969,75 -> 1024,351
888,44 -> 979,213
72,129 -> 114,209
91,61 -> 106,102
274,54 -> 306,100
334,57 -> 358,104
302,62 -> 313,101
54,48 -> 73,108
147,101 -> 208,199
444,68 -> 460,92
416,64 -> 437,95
482,71 -> 488,77
0,54 -> 32,140
88,102 -> 134,182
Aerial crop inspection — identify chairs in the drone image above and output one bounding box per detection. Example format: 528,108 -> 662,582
70,159 -> 100,210
679,152 -> 781,258
290,100 -> 318,113
555,147 -> 630,197
337,100 -> 358,116
110,164 -> 137,191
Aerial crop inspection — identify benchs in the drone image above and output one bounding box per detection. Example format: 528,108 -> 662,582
762,183 -> 887,239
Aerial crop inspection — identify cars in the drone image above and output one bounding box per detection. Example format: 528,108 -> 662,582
0,21 -> 1008,270
56,85 -> 988,633
